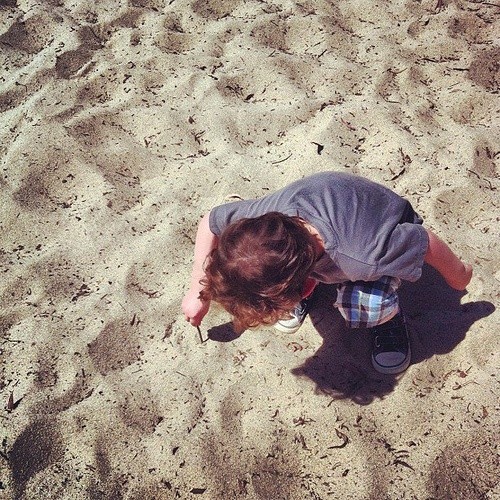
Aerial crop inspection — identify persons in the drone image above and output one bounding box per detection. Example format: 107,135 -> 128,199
176,167 -> 476,375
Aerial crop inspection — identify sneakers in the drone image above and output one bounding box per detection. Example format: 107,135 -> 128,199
369,302 -> 411,374
274,284 -> 316,333
